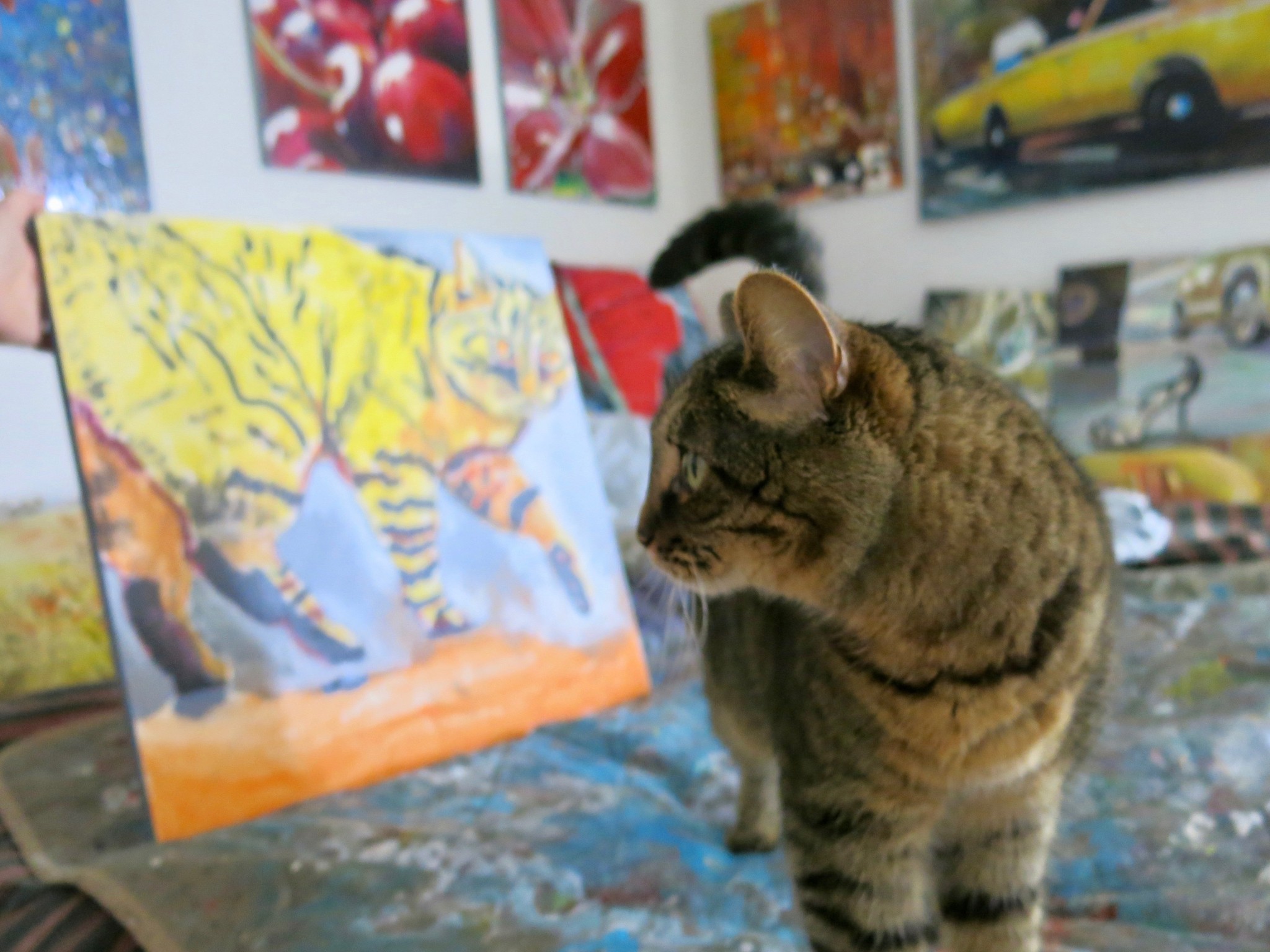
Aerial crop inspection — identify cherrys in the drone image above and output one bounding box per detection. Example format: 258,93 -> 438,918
245,1 -> 481,181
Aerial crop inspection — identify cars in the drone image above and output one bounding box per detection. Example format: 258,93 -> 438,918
1170,247 -> 1270,347
927,0 -> 1270,167
1072,431 -> 1270,568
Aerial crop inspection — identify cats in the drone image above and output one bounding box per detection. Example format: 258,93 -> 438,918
635,200 -> 1114,950
